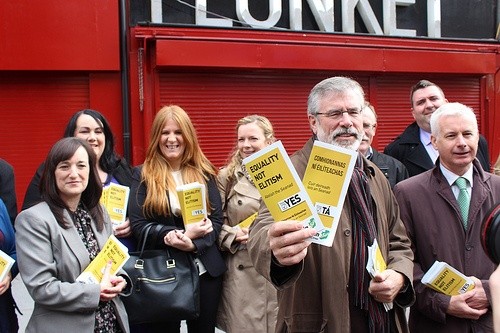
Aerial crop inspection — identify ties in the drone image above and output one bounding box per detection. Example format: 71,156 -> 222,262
455,177 -> 470,232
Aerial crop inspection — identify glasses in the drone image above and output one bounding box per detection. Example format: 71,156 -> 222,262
363,123 -> 376,130
317,109 -> 362,120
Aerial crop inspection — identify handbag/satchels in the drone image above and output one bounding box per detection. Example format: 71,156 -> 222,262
122,222 -> 200,320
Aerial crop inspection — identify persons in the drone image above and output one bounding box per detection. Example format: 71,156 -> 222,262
0,198 -> 22,333
15,137 -> 133,333
357,101 -> 410,188
246,77 -> 415,333
392,102 -> 500,332
129,105 -> 228,333
205,114 -> 278,333
384,80 -> 491,178
0,157 -> 18,232
488,264 -> 500,332
22,109 -> 131,252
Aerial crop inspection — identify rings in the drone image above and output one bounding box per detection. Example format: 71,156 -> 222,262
246,240 -> 247,245
180,234 -> 184,239
167,239 -> 171,242
